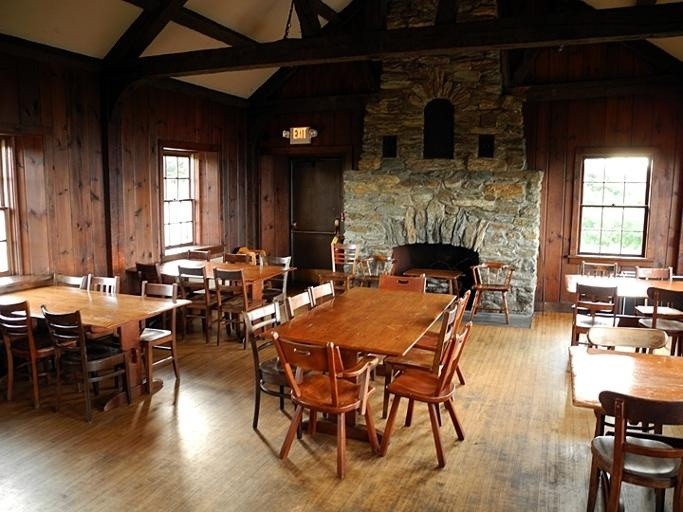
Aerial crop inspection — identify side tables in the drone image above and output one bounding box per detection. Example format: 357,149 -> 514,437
402,268 -> 462,300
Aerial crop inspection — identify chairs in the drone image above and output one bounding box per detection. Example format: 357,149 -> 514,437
586,390 -> 683,512
571,282 -> 621,351
357,255 -> 391,288
468,262 -> 516,325
1,300 -> 77,410
284,287 -> 314,320
633,266 -> 683,320
184,249 -> 211,289
247,254 -> 292,328
241,298 -> 328,440
85,273 -> 120,340
208,253 -> 250,296
53,273 -> 87,289
571,261 -> 618,327
177,265 -> 231,343
362,273 -> 427,381
586,326 -> 668,438
382,304 -> 457,427
134,260 -> 189,330
115,281 -> 181,395
40,304 -> 132,422
378,322 -> 471,467
271,331 -> 382,480
213,267 -> 266,350
318,242 -> 360,291
635,287 -> 683,357
309,279 -> 336,307
414,289 -> 471,385
